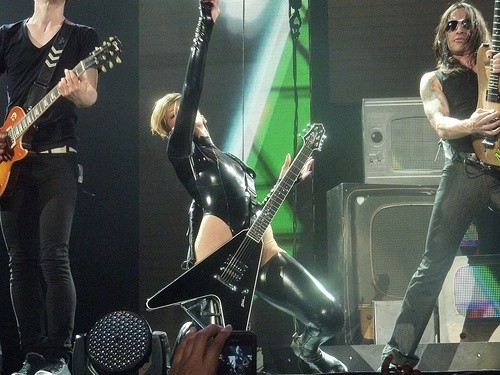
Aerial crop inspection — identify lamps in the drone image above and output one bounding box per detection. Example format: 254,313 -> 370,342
71,310 -> 170,375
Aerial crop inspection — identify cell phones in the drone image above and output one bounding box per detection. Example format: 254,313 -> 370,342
214,330 -> 257,375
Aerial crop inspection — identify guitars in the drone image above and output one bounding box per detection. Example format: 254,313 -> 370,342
146,123 -> 327,333
471,0 -> 500,166
0,36 -> 125,198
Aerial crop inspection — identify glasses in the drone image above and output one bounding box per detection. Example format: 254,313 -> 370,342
446,19 -> 471,32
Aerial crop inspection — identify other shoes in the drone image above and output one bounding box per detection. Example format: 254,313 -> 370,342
292,332 -> 348,374
10,358 -> 72,375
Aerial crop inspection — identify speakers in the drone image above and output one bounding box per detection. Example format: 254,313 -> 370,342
326,97 -> 500,348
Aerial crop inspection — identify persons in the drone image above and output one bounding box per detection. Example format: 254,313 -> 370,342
150,0 -> 350,375
0,0 -> 98,375
378,2 -> 500,375
168,324 -> 232,375
